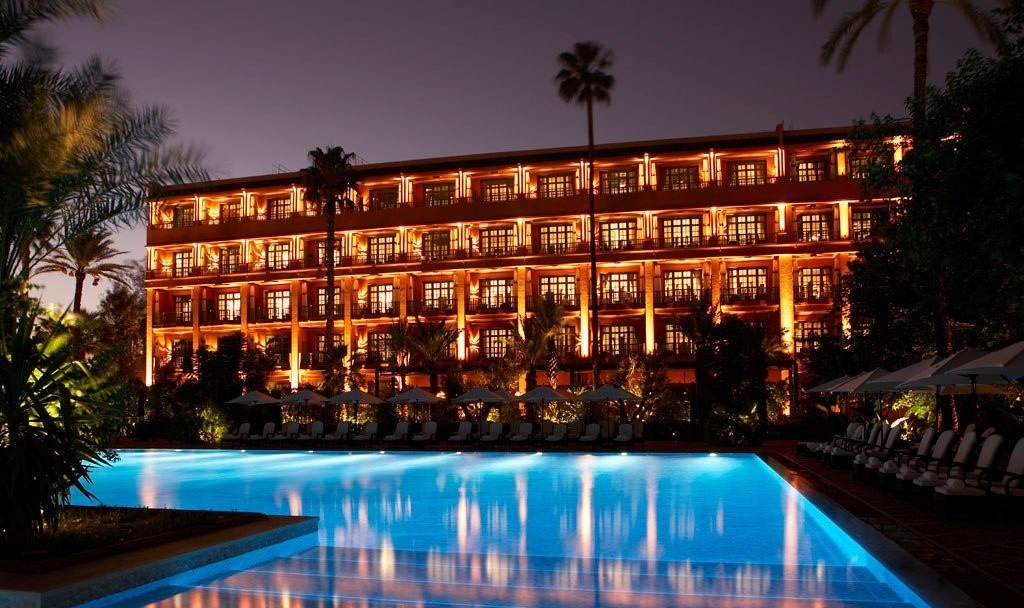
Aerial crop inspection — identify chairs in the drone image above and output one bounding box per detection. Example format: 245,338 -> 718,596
220,420 -> 644,451
797,422 -> 1024,515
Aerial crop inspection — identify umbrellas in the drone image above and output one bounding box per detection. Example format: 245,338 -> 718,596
807,340 -> 1024,454
278,388 -> 329,417
580,383 -> 640,437
225,390 -> 280,407
386,386 -> 445,423
513,385 -> 571,436
451,386 -> 507,436
329,389 -> 384,420
